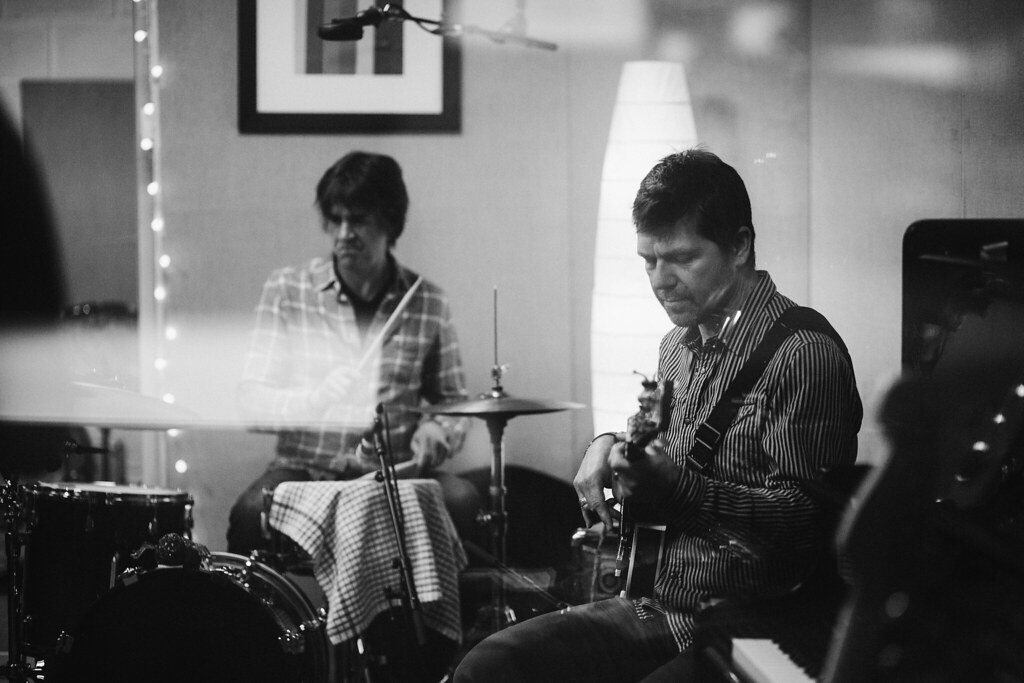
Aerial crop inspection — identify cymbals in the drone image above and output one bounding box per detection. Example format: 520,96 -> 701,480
419,390 -> 584,417
7,440 -> 112,457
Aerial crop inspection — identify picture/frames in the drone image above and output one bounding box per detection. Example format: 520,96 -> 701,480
235,0 -> 463,133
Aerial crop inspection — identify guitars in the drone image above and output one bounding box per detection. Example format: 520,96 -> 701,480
576,371 -> 677,597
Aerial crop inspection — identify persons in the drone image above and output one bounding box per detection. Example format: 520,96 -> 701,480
456,150 -> 864,683
228,152 -> 470,562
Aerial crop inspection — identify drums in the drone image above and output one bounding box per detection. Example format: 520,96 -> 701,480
43,551 -> 359,683
22,479 -> 193,649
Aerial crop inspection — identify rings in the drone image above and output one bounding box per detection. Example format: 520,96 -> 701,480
613,472 -> 619,481
581,501 -> 587,507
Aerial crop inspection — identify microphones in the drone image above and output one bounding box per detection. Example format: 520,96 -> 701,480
318,20 -> 364,41
